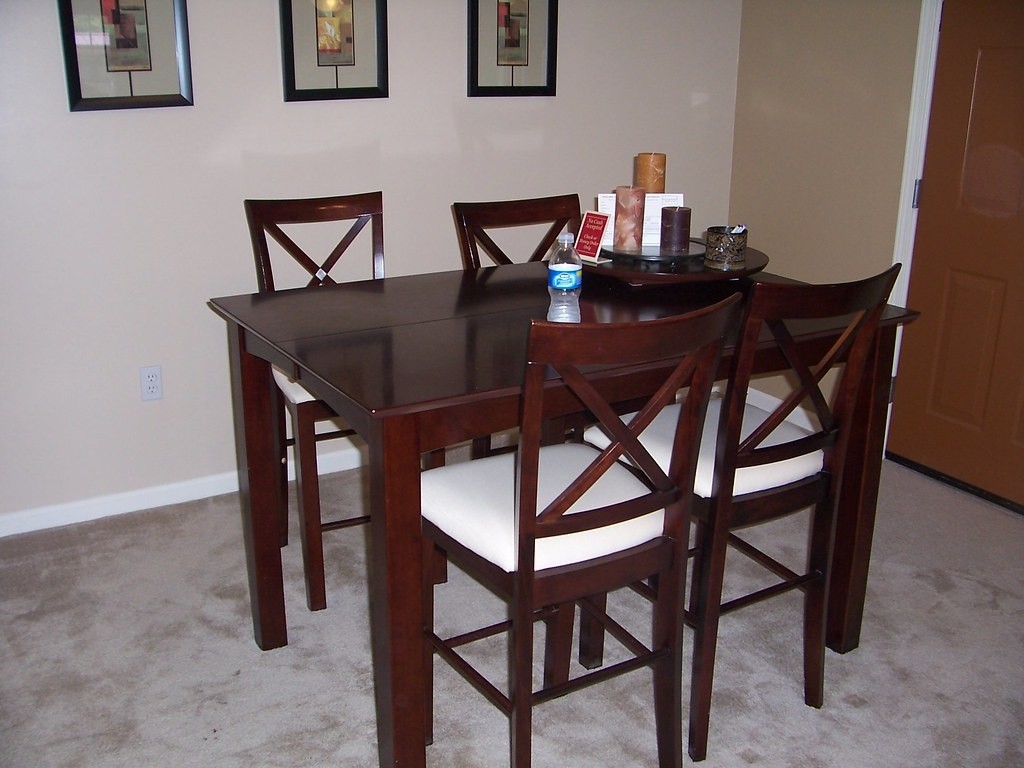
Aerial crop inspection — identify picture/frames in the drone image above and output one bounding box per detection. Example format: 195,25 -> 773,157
279,0 -> 391,104
58,0 -> 195,114
466,0 -> 559,97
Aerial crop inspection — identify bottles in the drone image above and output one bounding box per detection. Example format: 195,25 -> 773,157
546,232 -> 584,323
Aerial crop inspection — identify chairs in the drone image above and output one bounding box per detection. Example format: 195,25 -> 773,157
421,286 -> 744,768
448,189 -> 597,457
577,260 -> 902,764
239,188 -> 449,612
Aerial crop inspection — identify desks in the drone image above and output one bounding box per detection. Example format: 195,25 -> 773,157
201,245 -> 924,768
